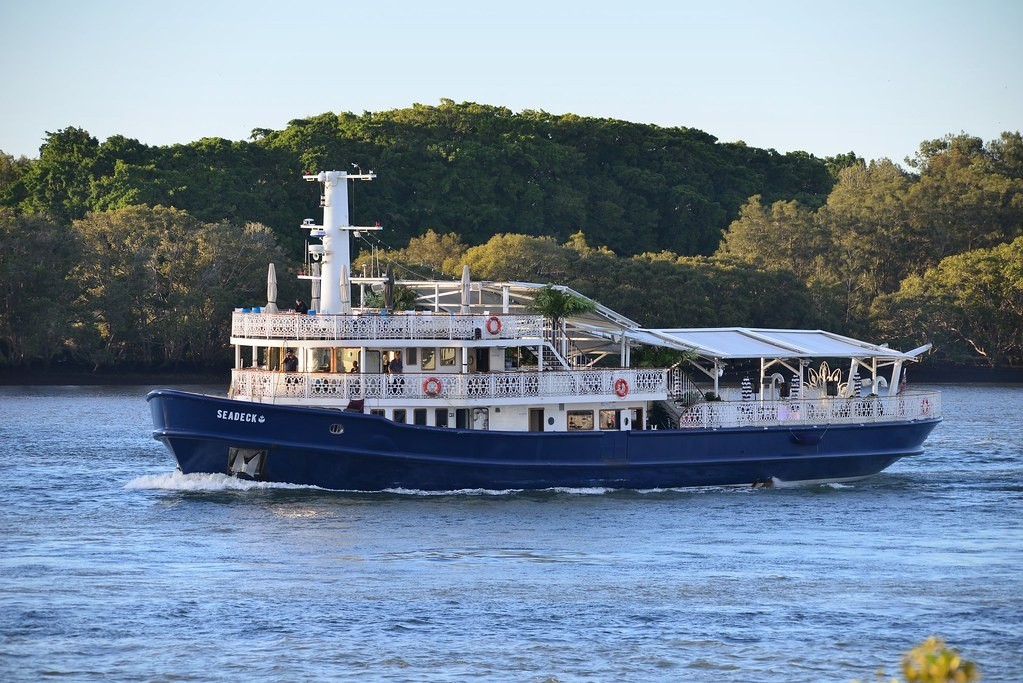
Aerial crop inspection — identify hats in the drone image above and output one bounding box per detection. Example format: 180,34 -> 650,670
287,349 -> 293,352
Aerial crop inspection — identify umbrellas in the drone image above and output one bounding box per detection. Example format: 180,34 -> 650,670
311,263 -> 320,298
384,264 -> 394,306
267,263 -> 278,301
461,264 -> 470,305
339,264 -> 350,303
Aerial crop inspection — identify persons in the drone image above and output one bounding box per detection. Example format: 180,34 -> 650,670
383,353 -> 402,374
324,350 -> 329,364
281,349 -> 298,373
607,414 -> 615,427
291,299 -> 308,314
350,361 -> 360,373
570,417 -> 588,429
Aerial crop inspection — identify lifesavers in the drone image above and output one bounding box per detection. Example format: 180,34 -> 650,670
423,377 -> 442,396
487,316 -> 502,334
615,378 -> 629,397
921,398 -> 929,414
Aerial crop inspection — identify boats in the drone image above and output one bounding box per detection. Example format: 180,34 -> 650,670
146,163 -> 943,493
